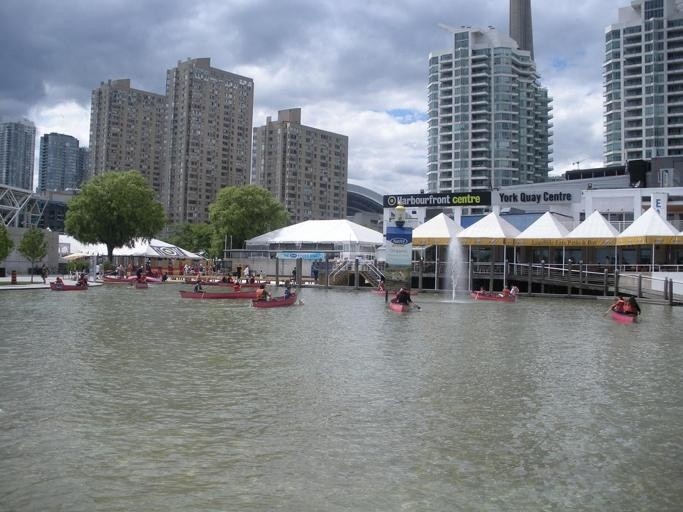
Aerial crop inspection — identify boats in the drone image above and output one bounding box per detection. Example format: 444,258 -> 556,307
604,303 -> 646,325
371,286 -> 418,296
471,290 -> 520,303
50,262 -> 304,308
388,293 -> 410,314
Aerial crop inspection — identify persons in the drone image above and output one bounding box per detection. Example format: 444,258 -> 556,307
378,281 -> 413,305
608,295 -> 641,317
498,283 -> 519,298
75,257 -> 323,301
55,277 -> 63,284
